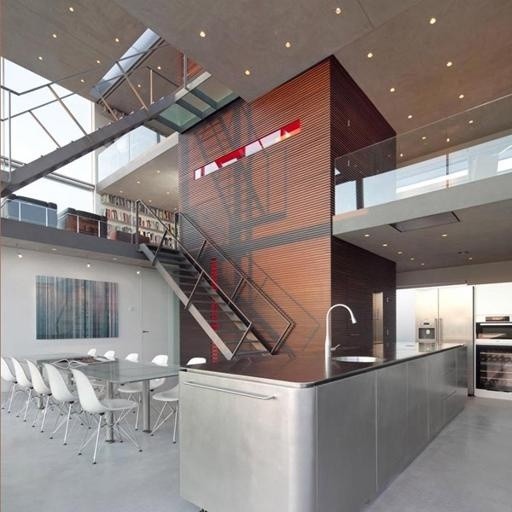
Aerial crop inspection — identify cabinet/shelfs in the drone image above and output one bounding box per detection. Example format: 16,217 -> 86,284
102,201 -> 177,248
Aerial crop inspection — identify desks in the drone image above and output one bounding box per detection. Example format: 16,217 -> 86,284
38,358 -> 184,434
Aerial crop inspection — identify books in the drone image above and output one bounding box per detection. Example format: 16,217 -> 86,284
101,194 -> 174,247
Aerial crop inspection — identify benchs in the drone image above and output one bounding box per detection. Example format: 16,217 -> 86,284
111,231 -> 149,245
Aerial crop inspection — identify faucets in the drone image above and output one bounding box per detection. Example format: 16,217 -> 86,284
324,302 -> 357,358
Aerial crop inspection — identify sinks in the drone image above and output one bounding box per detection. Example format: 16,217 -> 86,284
335,355 -> 383,364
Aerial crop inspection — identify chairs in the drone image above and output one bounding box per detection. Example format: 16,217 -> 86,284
1,357 -> 142,462
90,348 -> 206,444
